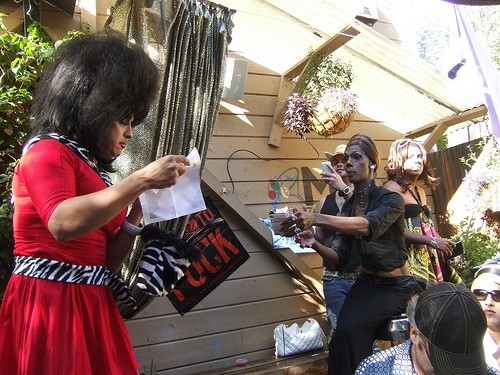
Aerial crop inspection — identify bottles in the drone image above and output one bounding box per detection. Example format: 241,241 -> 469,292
389,316 -> 411,347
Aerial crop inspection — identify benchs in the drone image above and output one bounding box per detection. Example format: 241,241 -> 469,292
196,349 -> 329,375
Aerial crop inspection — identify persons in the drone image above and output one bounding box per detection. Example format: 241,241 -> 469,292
470,263 -> 500,371
354,282 -> 500,375
278,133 -> 426,375
295,144 -> 366,344
0,29 -> 190,373
381,138 -> 456,283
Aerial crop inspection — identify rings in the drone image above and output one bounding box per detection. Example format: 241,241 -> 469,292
290,214 -> 298,221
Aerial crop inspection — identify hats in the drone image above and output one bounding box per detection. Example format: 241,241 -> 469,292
414,282 -> 488,375
324,144 -> 348,161
346,132 -> 380,171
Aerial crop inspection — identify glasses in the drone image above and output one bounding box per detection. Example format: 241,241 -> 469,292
398,138 -> 418,154
473,288 -> 500,301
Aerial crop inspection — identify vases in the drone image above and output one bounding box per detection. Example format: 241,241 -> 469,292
304,109 -> 355,137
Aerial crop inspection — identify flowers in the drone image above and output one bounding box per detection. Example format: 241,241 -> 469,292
281,45 -> 360,138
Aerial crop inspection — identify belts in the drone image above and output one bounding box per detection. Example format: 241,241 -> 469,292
323,269 -> 360,279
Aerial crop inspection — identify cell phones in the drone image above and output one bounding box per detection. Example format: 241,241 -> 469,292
442,241 -> 463,262
322,162 -> 331,182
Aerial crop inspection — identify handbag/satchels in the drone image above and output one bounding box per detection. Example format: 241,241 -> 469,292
273,317 -> 327,358
135,215 -> 224,298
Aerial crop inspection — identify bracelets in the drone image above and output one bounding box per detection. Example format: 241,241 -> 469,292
431,238 -> 439,250
121,219 -> 143,235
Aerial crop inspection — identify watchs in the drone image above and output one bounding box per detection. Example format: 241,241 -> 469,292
337,187 -> 352,196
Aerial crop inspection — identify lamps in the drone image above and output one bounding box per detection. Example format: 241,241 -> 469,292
223,58 -> 249,100
